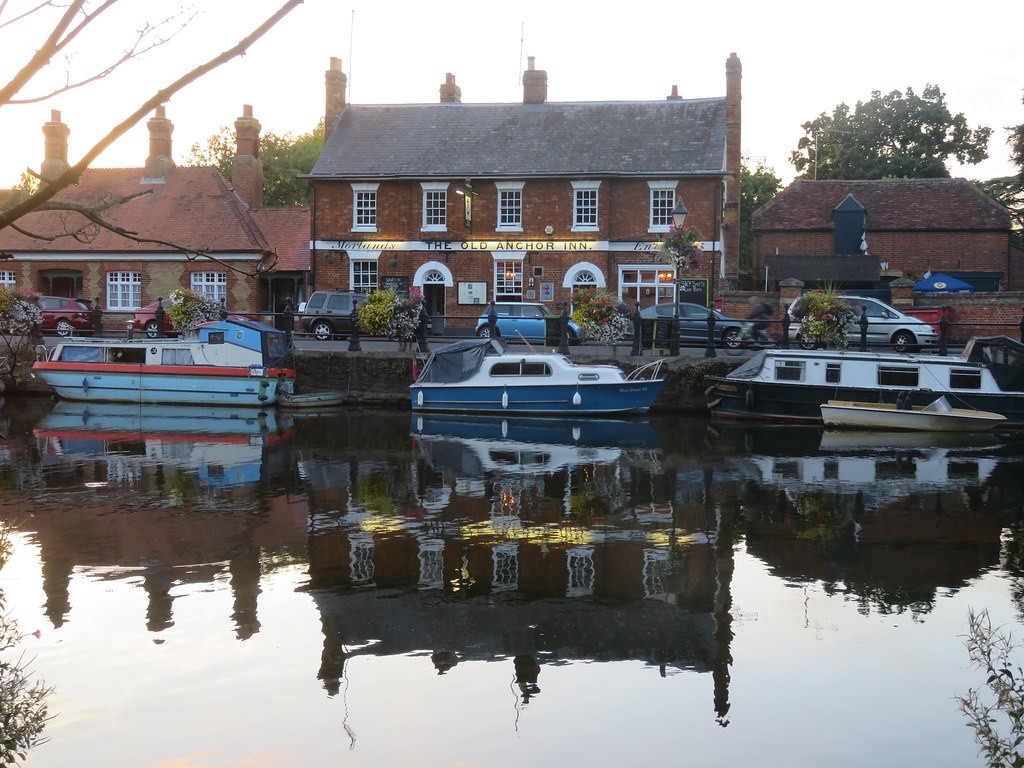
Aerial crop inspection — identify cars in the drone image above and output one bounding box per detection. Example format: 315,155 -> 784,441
632,302 -> 778,349
473,301 -> 586,345
133,299 -> 259,340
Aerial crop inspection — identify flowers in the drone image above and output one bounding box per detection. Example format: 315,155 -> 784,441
5,300 -> 44,337
656,224 -> 705,277
168,287 -> 221,334
789,280 -> 863,349
351,283 -> 426,339
568,282 -> 636,346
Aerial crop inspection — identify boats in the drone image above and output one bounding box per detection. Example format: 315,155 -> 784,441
818,398 -> 1008,436
407,339 -> 668,415
32,322 -> 295,409
703,346 -> 1024,430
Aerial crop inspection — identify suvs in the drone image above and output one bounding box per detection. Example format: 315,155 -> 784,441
787,292 -> 939,354
28,295 -> 97,338
296,290 -> 432,340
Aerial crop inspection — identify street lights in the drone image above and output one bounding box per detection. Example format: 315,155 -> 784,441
667,195 -> 692,356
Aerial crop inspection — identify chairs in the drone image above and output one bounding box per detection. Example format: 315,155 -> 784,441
896,391 -> 904,409
904,390 -> 913,410
